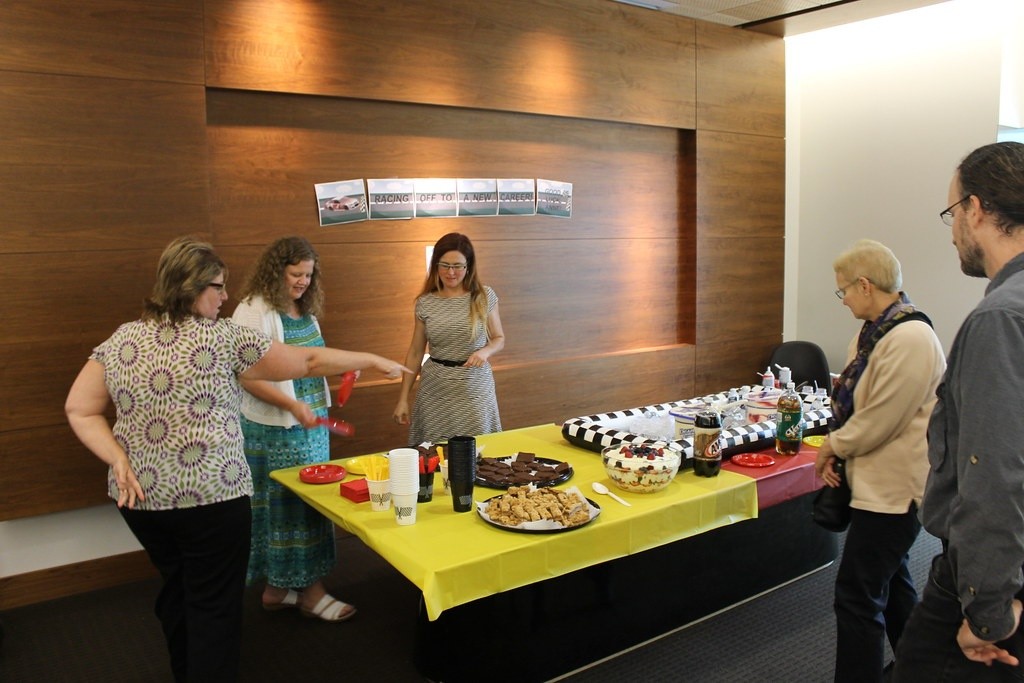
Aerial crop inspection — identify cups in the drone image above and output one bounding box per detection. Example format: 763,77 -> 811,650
800,386 -> 814,395
417,464 -> 434,503
814,389 -> 828,398
447,435 -> 476,512
365,476 -> 390,511
388,448 -> 420,526
439,459 -> 452,495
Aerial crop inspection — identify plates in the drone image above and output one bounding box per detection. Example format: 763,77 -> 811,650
730,452 -> 776,467
474,456 -> 574,491
803,436 -> 826,447
299,464 -> 346,485
477,493 -> 600,534
345,455 -> 389,475
338,372 -> 355,406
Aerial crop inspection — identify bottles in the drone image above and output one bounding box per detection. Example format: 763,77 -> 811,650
728,388 -> 739,403
775,363 -> 792,390
775,382 -> 803,456
740,385 -> 750,401
692,412 -> 722,477
762,366 -> 775,388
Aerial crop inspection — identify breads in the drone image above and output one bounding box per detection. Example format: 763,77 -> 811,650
487,485 -> 590,528
474,452 -> 570,484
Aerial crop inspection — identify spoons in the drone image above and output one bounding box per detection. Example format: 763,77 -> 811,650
592,481 -> 632,507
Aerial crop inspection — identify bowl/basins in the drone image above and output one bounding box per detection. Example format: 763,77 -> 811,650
601,443 -> 682,495
668,404 -> 695,439
746,404 -> 777,425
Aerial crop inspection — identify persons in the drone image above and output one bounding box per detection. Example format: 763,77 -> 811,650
65,233 -> 415,683
393,232 -> 505,447
231,236 -> 360,621
892,141 -> 1024,683
814,238 -> 947,683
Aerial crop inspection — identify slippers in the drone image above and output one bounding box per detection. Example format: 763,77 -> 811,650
262,588 -> 299,609
300,594 -> 357,621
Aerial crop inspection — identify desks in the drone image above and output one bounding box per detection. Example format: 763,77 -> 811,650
269,387 -> 839,683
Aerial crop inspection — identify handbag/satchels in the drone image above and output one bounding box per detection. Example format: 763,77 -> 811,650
812,458 -> 852,531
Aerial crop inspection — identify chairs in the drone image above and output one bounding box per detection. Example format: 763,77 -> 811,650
760,342 -> 831,397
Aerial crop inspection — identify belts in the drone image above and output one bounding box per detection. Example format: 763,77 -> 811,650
429,356 -> 466,368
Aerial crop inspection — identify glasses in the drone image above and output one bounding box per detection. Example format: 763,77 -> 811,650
206,283 -> 226,295
836,277 -> 872,299
435,262 -> 469,272
940,193 -> 989,227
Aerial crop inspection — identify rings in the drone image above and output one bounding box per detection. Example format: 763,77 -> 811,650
118,488 -> 128,493
393,374 -> 397,377
